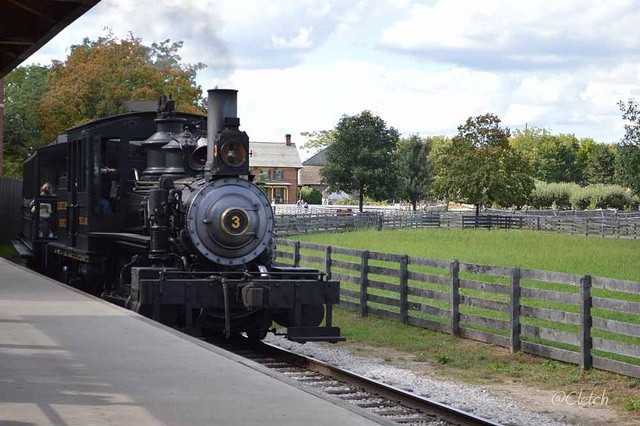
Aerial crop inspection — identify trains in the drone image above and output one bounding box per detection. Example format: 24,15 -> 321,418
12,89 -> 345,343
